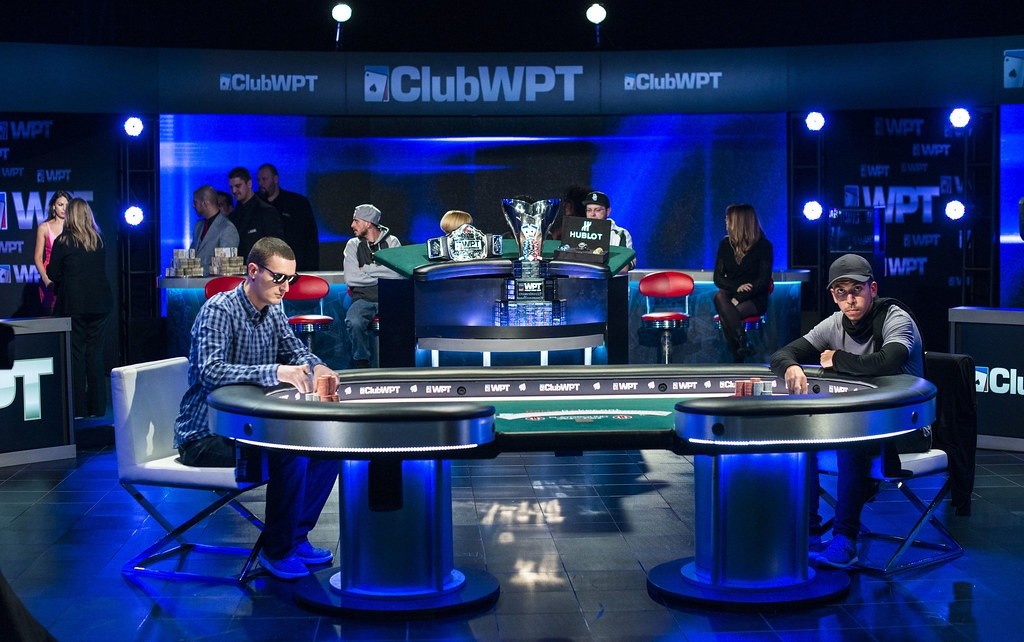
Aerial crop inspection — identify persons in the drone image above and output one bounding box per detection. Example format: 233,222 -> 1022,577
190,185 -> 239,277
217,192 -> 234,215
254,164 -> 319,272
770,254 -> 924,563
173,237 -> 340,580
583,191 -> 636,274
440,210 -> 472,234
343,205 -> 402,367
33,189 -> 112,419
229,167 -> 285,265
713,203 -> 772,361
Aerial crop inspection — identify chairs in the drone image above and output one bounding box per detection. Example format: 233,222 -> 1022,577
279,275 -> 334,353
713,278 -> 774,331
808,351 -> 979,581
638,272 -> 694,364
110,357 -> 270,588
204,275 -> 250,301
346,286 -> 380,333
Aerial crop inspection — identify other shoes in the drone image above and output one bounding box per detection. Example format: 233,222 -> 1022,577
257,550 -> 311,581
737,337 -> 758,356
291,538 -> 336,566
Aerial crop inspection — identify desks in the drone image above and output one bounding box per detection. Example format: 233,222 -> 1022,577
206,364 -> 937,620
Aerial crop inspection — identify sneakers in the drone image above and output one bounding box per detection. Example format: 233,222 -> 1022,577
814,534 -> 861,567
809,541 -> 831,560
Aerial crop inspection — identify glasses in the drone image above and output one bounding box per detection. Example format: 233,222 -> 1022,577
256,263 -> 298,285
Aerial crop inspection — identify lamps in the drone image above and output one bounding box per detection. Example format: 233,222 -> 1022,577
584,0 -> 609,42
331,0 -> 352,48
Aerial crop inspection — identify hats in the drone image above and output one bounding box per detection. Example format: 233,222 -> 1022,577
581,192 -> 610,208
823,253 -> 875,286
354,203 -> 382,227
